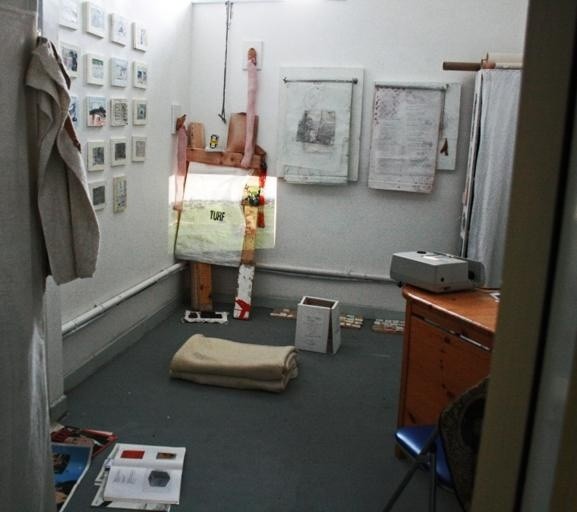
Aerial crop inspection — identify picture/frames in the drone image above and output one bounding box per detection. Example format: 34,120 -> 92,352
57,0 -> 148,215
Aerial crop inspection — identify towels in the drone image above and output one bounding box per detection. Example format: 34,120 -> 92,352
23,34 -> 99,284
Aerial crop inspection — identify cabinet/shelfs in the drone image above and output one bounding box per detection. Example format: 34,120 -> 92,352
394,284 -> 501,462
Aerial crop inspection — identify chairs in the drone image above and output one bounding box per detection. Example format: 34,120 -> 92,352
382,373 -> 490,511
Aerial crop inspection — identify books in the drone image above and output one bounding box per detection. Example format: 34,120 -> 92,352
49,421 -> 186,511
